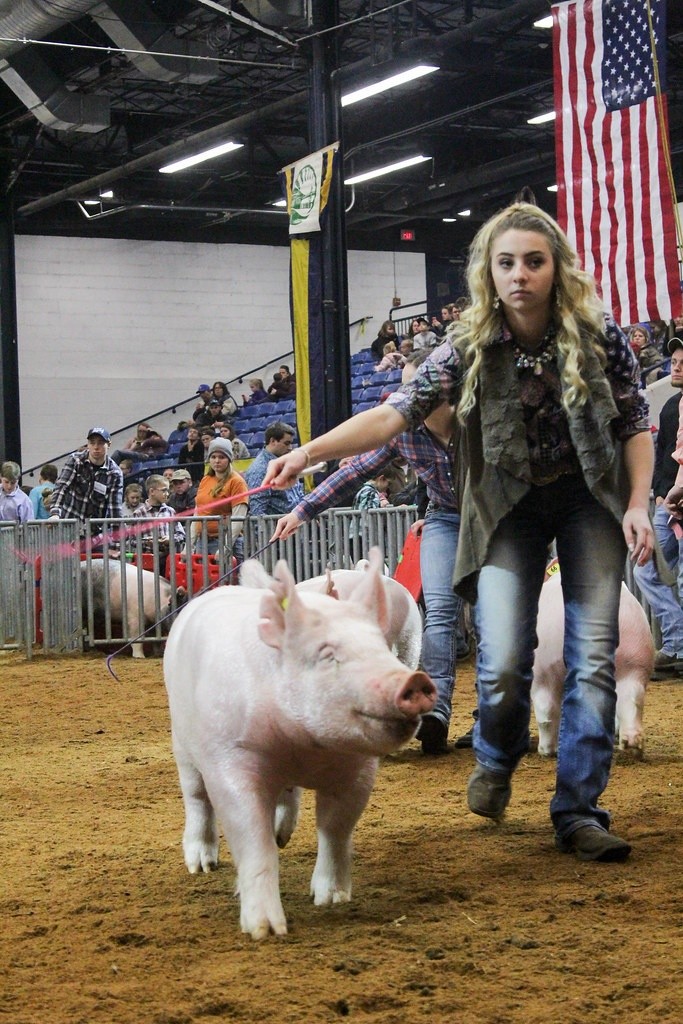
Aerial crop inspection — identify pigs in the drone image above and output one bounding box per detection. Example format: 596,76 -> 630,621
157,543 -> 438,942
78,557 -> 190,659
232,557 -> 425,671
531,567 -> 655,758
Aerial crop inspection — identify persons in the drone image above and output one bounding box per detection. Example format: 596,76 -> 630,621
163,469 -> 175,480
622,316 -> 683,669
122,483 -> 144,517
166,469 -> 198,514
47,428 -> 127,539
178,426 -> 250,482
42,487 -> 53,512
111,422 -> 170,475
242,364 -> 296,407
29,464 -> 58,520
127,474 -> 186,577
0,461 -> 34,522
244,420 -> 304,583
192,381 -> 240,436
371,296 -> 472,372
260,202 -> 655,861
347,463 -> 409,566
268,346 -> 479,754
181,437 -> 250,584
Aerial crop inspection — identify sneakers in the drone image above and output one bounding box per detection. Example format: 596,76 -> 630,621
467,765 -> 511,817
554,825 -> 632,861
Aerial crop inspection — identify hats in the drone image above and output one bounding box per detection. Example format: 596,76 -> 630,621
87,427 -> 111,442
170,469 -> 191,483
196,384 -> 210,394
208,437 -> 233,463
668,336 -> 683,354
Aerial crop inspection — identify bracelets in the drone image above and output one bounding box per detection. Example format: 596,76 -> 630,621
290,448 -> 310,470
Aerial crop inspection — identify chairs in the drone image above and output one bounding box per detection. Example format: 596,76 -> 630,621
121,331 -> 410,484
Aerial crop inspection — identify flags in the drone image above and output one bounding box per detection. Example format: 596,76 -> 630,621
551,0 -> 683,329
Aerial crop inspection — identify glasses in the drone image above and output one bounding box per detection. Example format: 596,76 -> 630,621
379,475 -> 392,486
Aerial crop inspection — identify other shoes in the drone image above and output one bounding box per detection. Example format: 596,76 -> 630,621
452,626 -> 477,661
455,723 -> 477,748
421,719 -> 449,754
651,649 -> 682,680
